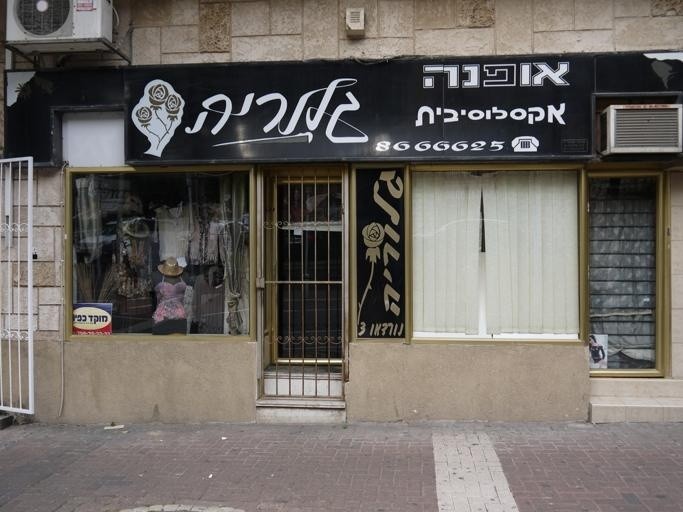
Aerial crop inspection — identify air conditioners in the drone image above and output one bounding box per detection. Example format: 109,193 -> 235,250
5,0 -> 113,55
597,104 -> 683,155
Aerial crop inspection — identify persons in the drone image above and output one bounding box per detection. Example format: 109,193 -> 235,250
150,272 -> 187,334
589,335 -> 607,369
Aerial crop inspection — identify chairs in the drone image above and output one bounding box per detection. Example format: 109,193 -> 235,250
195,275 -> 225,334
